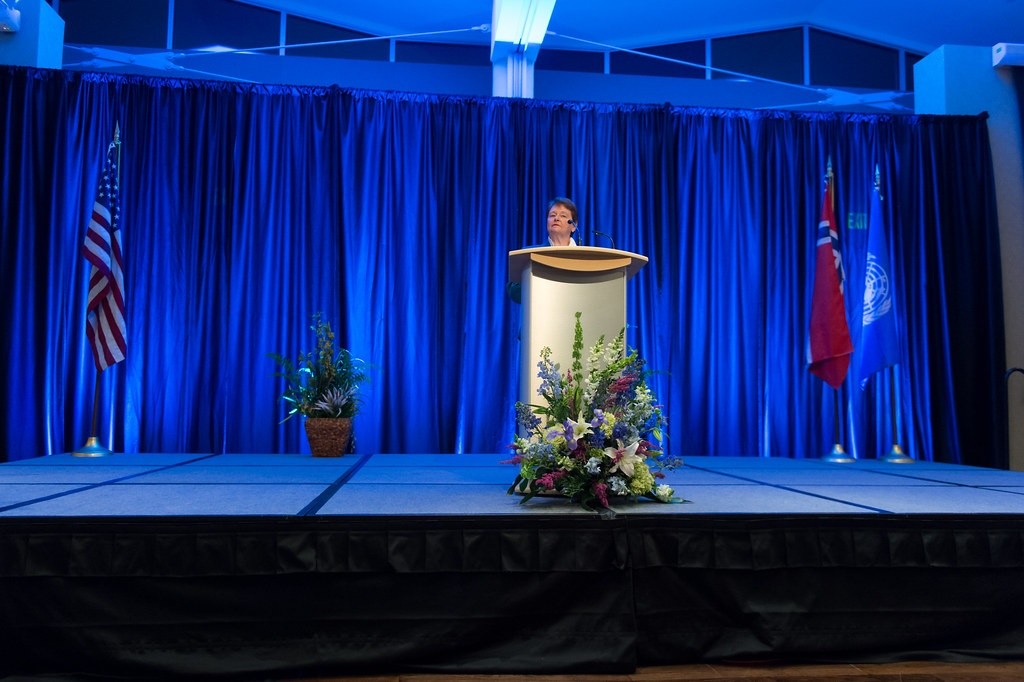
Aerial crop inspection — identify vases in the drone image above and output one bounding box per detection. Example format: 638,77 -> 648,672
304,414 -> 353,457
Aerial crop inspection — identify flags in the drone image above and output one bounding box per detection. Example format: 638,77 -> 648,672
82,136 -> 129,372
855,186 -> 902,390
805,176 -> 854,387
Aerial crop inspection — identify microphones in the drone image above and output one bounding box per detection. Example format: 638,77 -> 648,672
568,220 -> 581,246
594,231 -> 614,249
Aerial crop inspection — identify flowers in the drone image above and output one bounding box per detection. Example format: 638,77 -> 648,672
500,310 -> 694,513
264,313 -> 367,427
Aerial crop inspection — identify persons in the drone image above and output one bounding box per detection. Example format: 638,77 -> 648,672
508,197 -> 578,341
